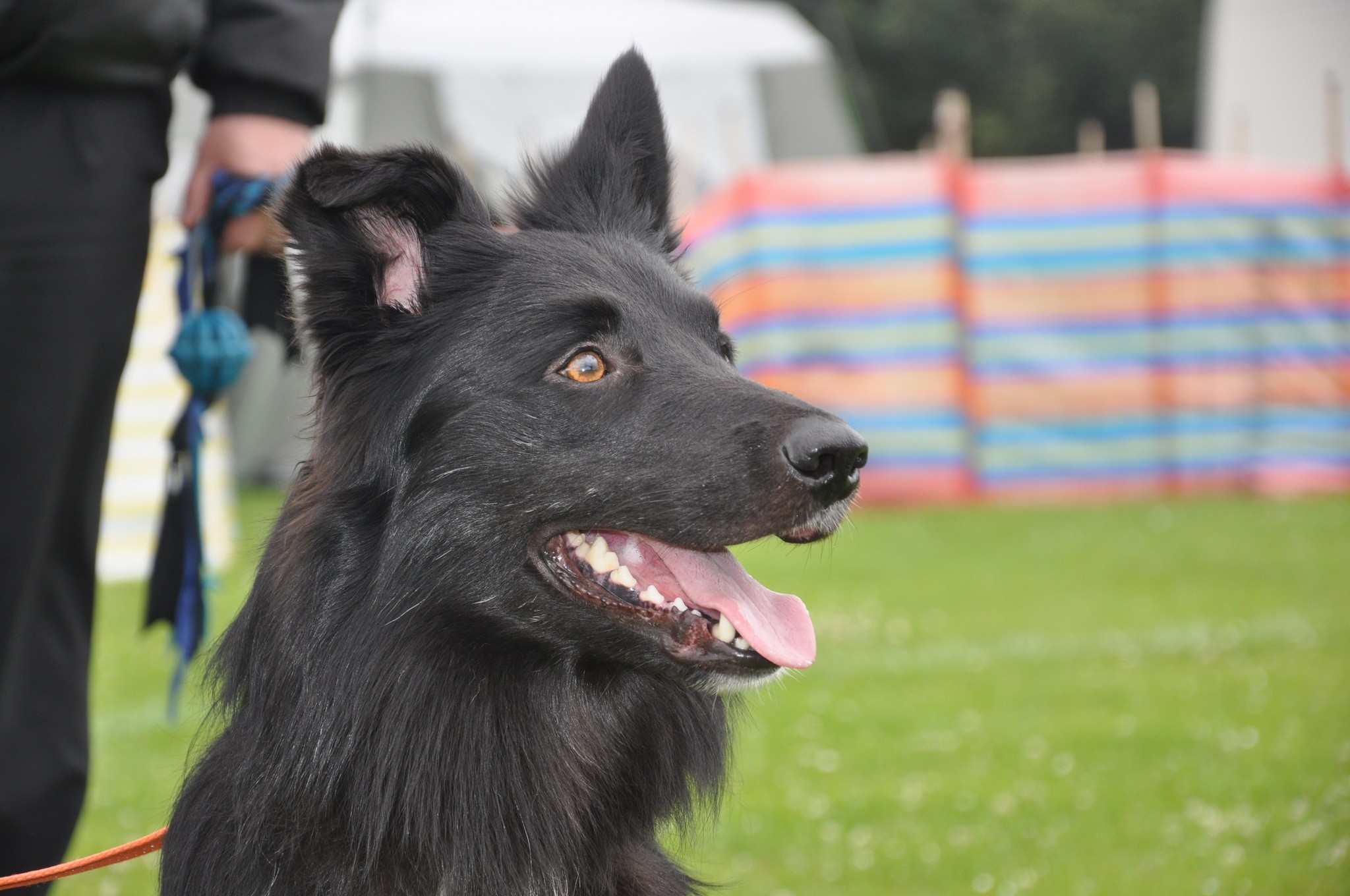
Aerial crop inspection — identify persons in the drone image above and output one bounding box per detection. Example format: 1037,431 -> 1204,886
0,0 -> 350,895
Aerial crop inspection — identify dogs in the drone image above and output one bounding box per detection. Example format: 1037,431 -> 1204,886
154,42 -> 869,896
